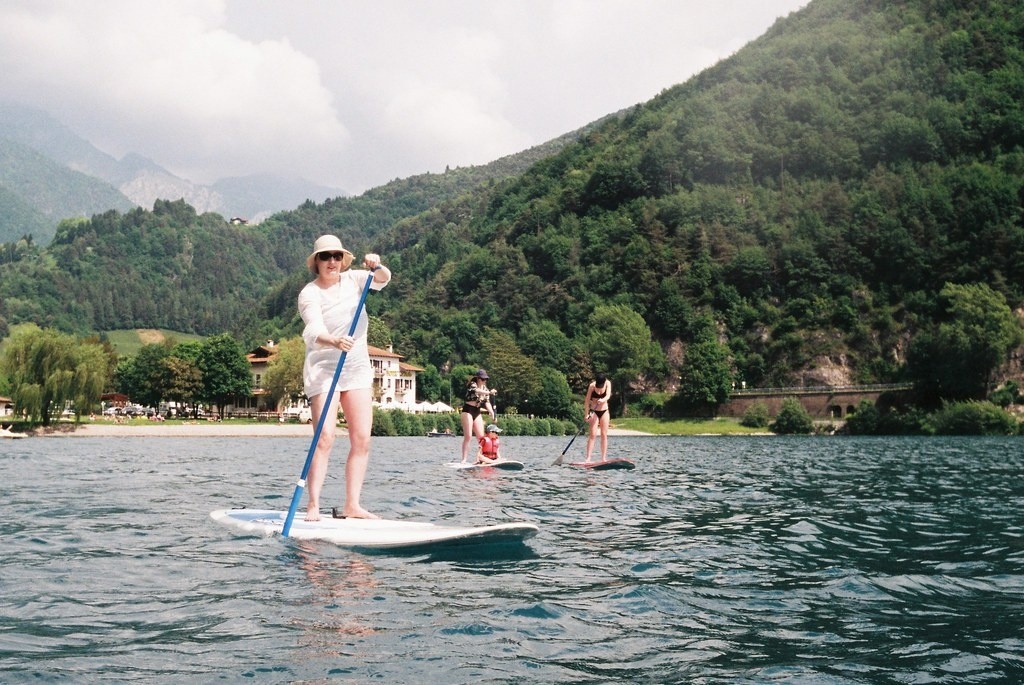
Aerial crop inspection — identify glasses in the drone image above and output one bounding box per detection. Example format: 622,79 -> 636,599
316,252 -> 343,261
481,378 -> 488,381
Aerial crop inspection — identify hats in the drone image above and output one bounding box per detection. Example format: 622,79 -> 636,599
486,424 -> 503,432
477,370 -> 489,379
307,235 -> 352,272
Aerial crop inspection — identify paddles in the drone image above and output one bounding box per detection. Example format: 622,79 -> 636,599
555,400 -> 601,464
491,391 -> 498,424
209,505 -> 542,557
280,262 -> 382,538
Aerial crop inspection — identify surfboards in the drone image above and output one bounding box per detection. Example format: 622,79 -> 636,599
444,460 -> 525,470
567,457 -> 636,470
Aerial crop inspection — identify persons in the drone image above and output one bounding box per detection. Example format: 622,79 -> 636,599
445,427 -> 451,434
584,372 -> 612,462
298,235 -> 392,522
473,424 -> 503,464
460,369 -> 497,464
